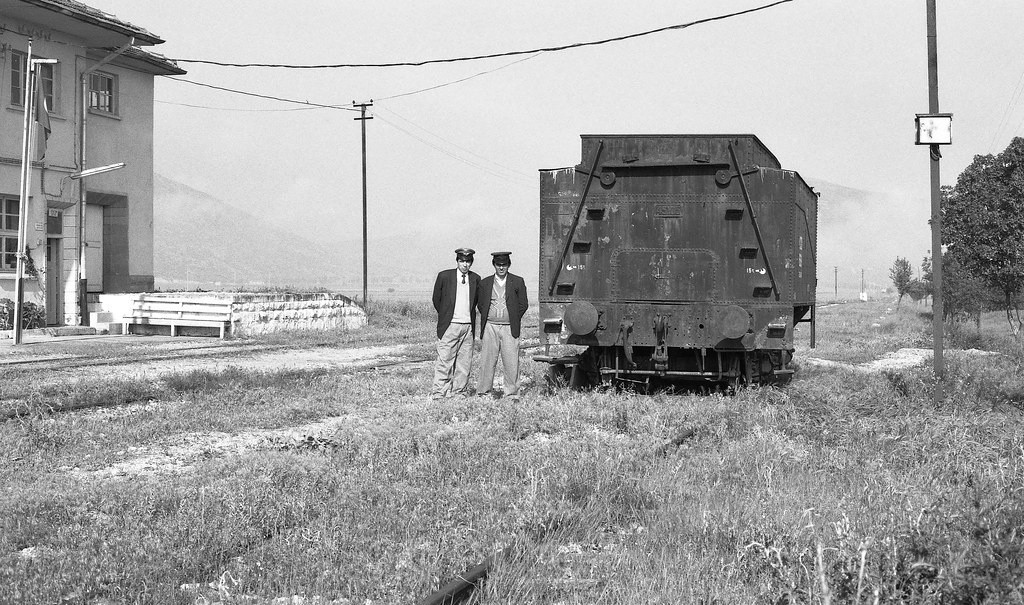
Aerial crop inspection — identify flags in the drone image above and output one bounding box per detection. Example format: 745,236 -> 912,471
34,74 -> 51,162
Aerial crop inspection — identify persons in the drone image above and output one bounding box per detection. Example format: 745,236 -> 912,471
477,252 -> 528,403
432,248 -> 481,399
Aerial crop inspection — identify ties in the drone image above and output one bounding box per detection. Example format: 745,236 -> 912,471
462,274 -> 466,284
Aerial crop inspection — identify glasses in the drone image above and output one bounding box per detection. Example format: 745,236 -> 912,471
494,263 -> 509,267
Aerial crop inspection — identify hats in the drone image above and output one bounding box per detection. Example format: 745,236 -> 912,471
455,248 -> 475,261
490,251 -> 512,264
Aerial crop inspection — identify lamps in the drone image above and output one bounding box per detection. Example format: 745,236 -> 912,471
69,162 -> 127,180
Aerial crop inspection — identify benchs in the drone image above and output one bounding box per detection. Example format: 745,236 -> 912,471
120,296 -> 234,339
